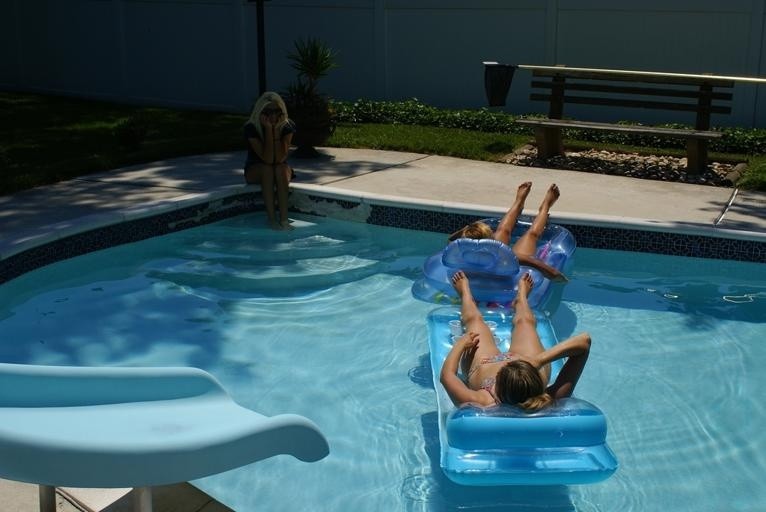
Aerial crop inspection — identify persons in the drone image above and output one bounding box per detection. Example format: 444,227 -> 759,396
439,269 -> 592,415
243,90 -> 296,231
447,181 -> 569,284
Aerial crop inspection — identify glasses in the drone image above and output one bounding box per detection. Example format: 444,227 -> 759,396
262,107 -> 282,117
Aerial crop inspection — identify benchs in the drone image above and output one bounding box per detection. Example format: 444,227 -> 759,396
510,64 -> 736,174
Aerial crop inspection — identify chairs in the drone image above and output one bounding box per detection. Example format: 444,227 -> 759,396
428,300 -> 623,490
411,212 -> 580,308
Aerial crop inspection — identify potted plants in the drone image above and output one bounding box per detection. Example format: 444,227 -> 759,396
268,37 -> 348,161
